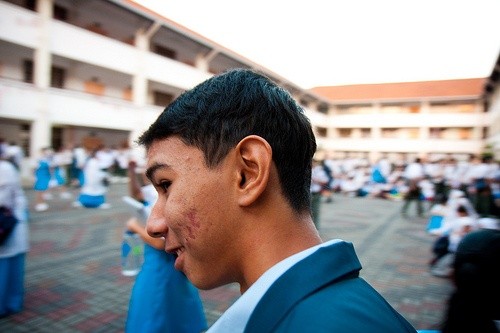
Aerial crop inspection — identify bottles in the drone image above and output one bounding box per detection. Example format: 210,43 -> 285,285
121,231 -> 145,277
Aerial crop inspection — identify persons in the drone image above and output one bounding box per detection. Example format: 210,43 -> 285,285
138,69 -> 417,333
0,157 -> 29,311
311,158 -> 329,232
0,138 -> 25,171
126,160 -> 208,333
30,142 -> 134,213
323,155 -> 500,275
440,197 -> 500,333
